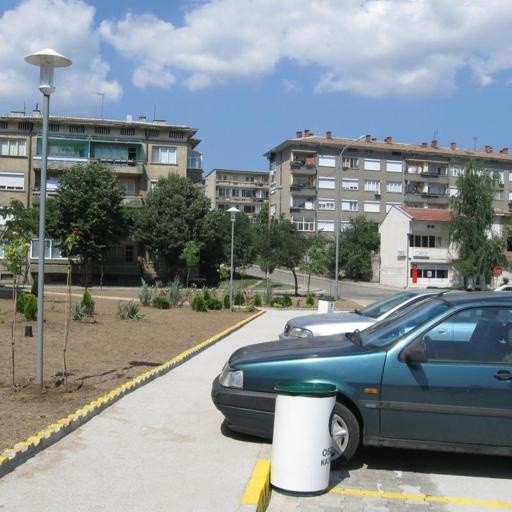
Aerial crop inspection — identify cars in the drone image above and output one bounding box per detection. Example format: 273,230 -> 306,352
211,291 -> 511,470
492,284 -> 511,292
278,289 -> 512,356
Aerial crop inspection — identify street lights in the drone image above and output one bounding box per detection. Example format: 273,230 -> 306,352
334,134 -> 372,300
25,46 -> 73,389
225,206 -> 241,312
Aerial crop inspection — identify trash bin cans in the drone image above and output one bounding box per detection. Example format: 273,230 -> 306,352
271,382 -> 337,496
317,296 -> 336,313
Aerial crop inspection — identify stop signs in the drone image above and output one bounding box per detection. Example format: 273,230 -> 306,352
493,266 -> 502,276
